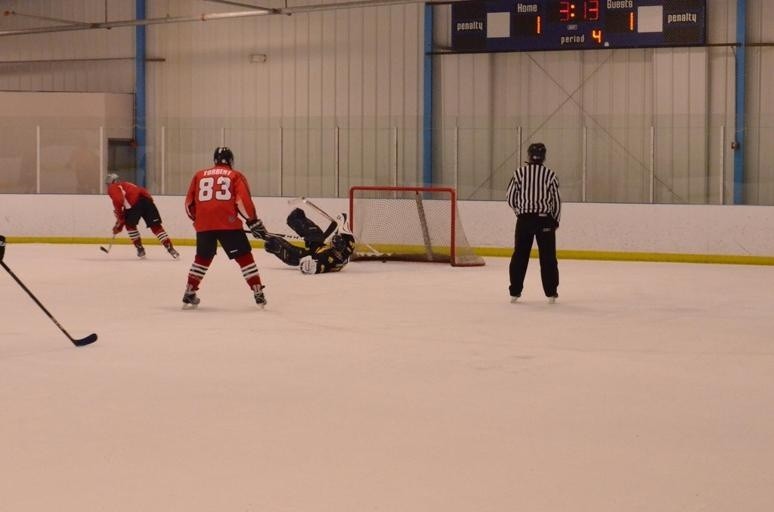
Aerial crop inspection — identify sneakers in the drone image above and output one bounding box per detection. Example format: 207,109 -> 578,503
509,287 -> 559,299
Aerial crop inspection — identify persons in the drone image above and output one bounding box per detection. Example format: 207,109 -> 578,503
105,171 -> 178,260
237,206 -> 355,274
179,143 -> 265,304
503,142 -> 562,298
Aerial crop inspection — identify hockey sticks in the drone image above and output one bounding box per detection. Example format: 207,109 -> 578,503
301,196 -> 388,264
100,234 -> 115,254
0,261 -> 97,346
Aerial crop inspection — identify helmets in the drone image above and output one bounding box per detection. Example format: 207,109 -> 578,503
105,172 -> 120,186
212,146 -> 233,167
527,142 -> 547,165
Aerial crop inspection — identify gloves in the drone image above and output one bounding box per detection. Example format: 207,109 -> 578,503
112,210 -> 126,234
245,220 -> 269,239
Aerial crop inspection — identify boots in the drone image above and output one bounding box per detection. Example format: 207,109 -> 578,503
164,243 -> 178,256
253,291 -> 268,305
182,291 -> 201,305
136,246 -> 146,257
